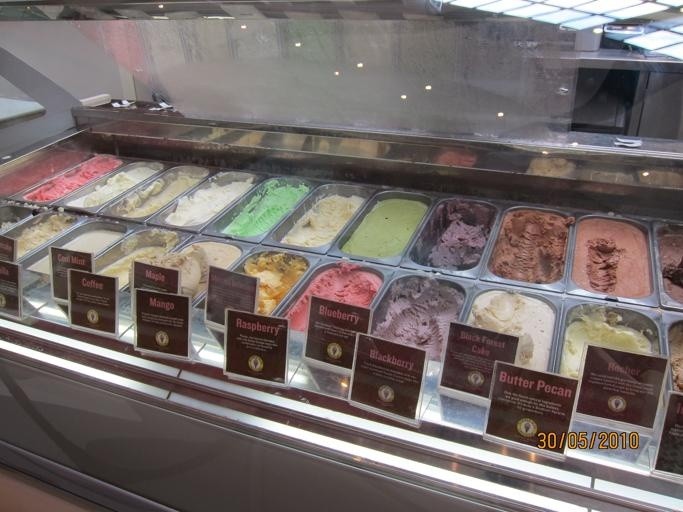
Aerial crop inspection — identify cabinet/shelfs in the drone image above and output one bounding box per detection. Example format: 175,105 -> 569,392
0,1 -> 682,511
